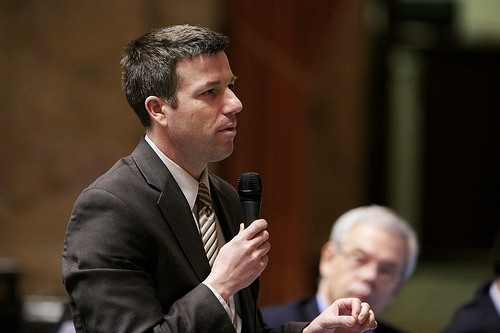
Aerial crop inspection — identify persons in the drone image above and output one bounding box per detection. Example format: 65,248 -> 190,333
58,24 -> 377,333
439,279 -> 500,333
258,204 -> 416,333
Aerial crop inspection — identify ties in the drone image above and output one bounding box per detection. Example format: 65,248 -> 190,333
195,183 -> 230,308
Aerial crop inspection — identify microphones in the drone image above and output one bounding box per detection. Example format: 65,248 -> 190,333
237,172 -> 264,301
